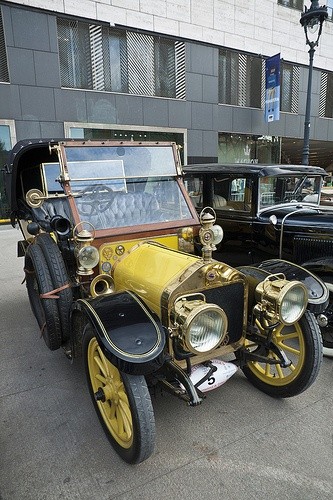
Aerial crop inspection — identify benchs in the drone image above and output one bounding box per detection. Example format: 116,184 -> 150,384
32,191 -> 161,298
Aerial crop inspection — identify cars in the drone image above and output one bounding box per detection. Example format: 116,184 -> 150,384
259,176 -> 333,203
7,139 -> 325,467
181,162 -> 333,347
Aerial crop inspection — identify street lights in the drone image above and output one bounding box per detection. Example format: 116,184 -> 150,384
298,0 -> 329,166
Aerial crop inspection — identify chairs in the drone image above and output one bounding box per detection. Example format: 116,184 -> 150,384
213,195 -> 234,210
